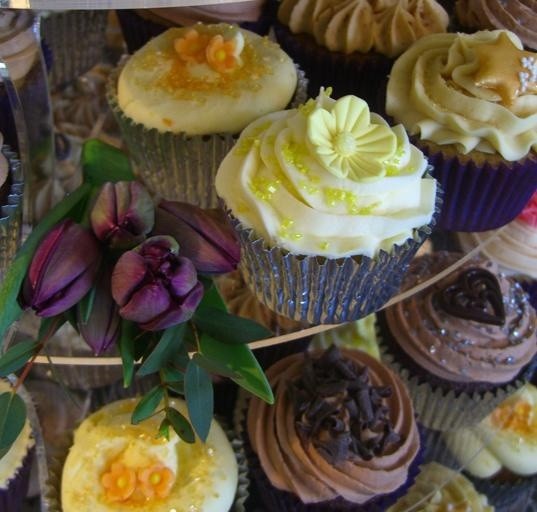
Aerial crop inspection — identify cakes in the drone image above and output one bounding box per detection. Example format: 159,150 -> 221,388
0,136 -> 25,277
106,21 -> 310,211
114,0 -> 277,56
384,29 -> 537,233
373,250 -> 537,434
0,9 -> 42,96
453,1 -> 536,51
276,0 -> 450,116
241,344 -> 424,507
214,88 -> 446,326
47,392 -> 246,512
